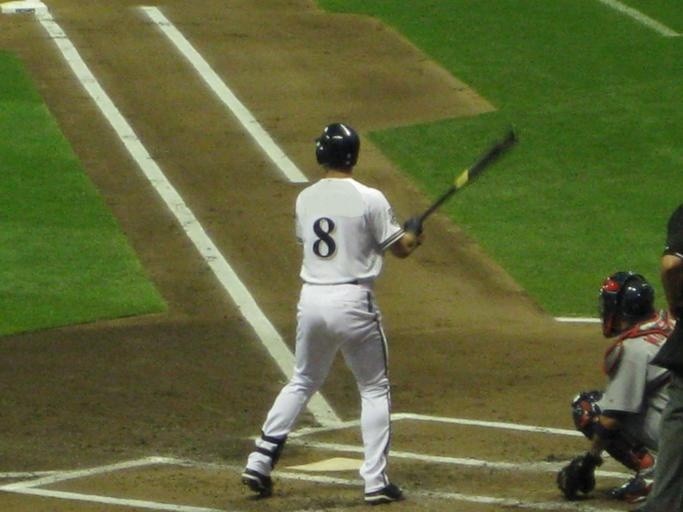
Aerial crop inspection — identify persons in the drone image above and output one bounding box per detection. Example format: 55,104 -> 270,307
554,270 -> 676,503
634,202 -> 682,512
241,122 -> 423,505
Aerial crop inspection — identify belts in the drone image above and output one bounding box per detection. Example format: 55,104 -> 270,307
303,279 -> 359,286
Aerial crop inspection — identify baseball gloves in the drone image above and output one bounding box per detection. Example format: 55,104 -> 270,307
556,456 -> 596,500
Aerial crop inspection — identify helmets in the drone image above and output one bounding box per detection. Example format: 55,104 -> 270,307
598,270 -> 656,338
315,122 -> 361,169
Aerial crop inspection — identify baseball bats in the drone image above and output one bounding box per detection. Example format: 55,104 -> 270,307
420,131 -> 518,220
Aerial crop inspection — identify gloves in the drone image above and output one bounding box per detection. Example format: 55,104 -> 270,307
404,216 -> 424,248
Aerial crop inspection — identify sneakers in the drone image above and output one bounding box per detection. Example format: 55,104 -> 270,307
239,466 -> 273,497
363,483 -> 409,504
608,467 -> 657,504
